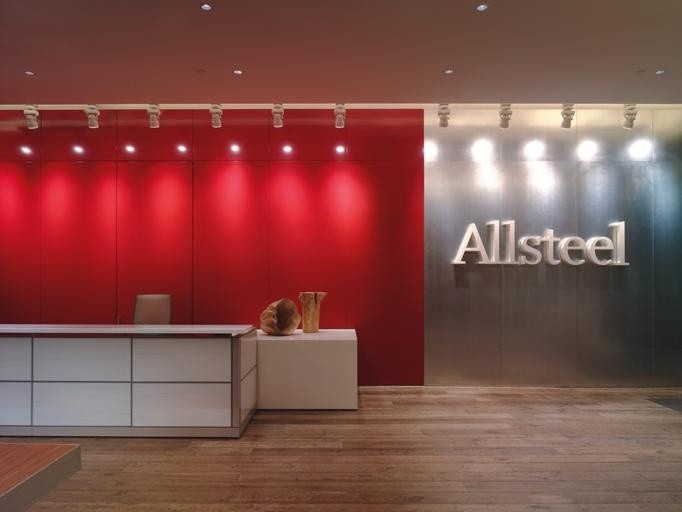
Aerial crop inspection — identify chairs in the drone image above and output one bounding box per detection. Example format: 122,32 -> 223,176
131,293 -> 174,326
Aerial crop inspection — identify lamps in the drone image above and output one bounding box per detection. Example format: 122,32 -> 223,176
20,103 -> 352,130
435,100 -> 640,131
